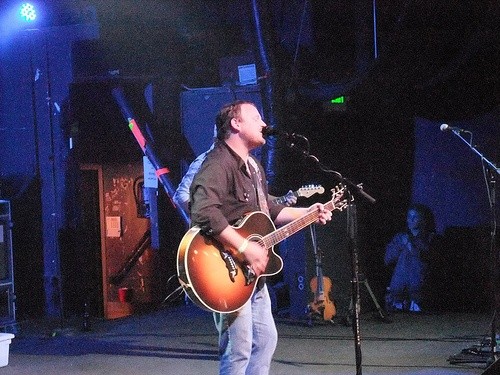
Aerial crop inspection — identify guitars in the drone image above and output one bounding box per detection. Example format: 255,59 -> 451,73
176,180 -> 363,315
307,225 -> 337,324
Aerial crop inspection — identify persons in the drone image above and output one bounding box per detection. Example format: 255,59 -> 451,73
171,113 -> 300,224
384,203 -> 445,313
189,100 -> 332,375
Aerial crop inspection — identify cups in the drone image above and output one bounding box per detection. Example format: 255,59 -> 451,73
119,288 -> 129,302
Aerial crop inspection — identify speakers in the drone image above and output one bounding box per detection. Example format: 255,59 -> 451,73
286,205 -> 381,319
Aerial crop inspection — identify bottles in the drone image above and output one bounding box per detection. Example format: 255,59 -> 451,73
83,303 -> 91,331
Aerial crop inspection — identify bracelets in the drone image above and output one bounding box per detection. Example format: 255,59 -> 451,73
231,238 -> 248,259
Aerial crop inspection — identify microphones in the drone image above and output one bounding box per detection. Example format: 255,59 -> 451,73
440,124 -> 465,133
263,125 -> 299,139
